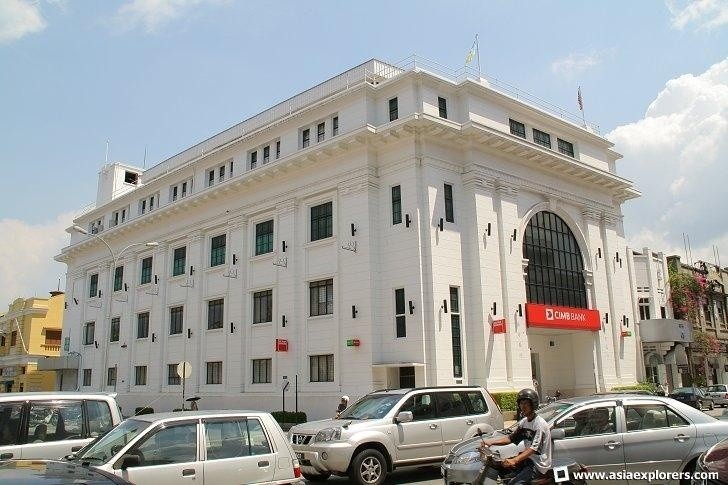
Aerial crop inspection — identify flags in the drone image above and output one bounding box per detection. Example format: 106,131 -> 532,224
465,38 -> 476,65
576,87 -> 583,111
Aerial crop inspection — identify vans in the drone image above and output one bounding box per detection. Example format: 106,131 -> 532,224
64,408 -> 308,484
0,390 -> 127,461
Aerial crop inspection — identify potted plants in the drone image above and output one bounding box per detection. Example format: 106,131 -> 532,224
267,410 -> 308,432
492,392 -> 518,421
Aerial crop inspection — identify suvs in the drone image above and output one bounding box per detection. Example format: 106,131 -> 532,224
285,384 -> 505,485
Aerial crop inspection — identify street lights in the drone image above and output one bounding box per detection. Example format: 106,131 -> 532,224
73,224 -> 161,394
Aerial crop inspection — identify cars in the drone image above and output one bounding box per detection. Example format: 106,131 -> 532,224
0,456 -> 133,485
667,385 -> 728,411
440,388 -> 728,485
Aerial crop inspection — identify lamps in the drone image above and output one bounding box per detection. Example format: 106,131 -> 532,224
64,213 -> 629,350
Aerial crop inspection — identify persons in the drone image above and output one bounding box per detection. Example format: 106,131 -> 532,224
655,384 -> 666,397
336,394 -> 349,415
478,387 -> 555,485
588,406 -> 615,435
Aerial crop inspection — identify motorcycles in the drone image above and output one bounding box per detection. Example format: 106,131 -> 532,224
471,427 -> 589,485
15,405 -> 58,427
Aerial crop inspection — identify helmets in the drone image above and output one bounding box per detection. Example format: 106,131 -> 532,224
517,389 -> 539,409
